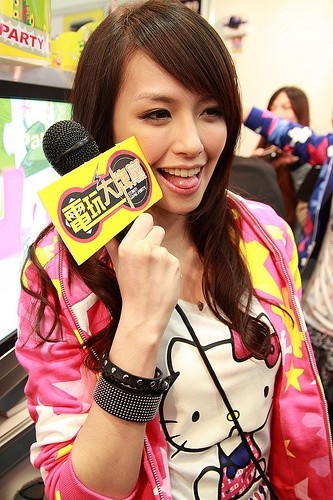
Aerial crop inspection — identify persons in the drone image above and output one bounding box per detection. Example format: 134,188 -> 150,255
240,104 -> 333,449
14,0 -> 333,500
1,98 -> 62,250
248,84 -> 322,254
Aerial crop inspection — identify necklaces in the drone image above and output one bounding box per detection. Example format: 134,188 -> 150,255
178,296 -> 208,311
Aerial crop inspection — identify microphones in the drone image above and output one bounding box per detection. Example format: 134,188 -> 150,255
42,120 -> 133,245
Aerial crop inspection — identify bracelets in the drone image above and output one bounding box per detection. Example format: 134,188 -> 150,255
92,346 -> 180,422
